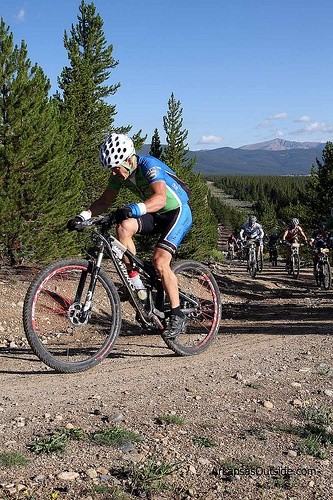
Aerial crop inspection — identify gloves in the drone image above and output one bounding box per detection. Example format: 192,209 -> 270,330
67,216 -> 85,233
112,207 -> 133,225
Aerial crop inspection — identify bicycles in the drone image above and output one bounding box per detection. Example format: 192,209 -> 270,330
285,239 -> 307,280
267,245 -> 278,267
228,244 -> 246,264
238,238 -> 266,277
313,246 -> 332,291
23,209 -> 222,374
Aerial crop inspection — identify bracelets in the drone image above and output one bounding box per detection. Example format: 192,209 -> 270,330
80,210 -> 92,220
136,203 -> 147,215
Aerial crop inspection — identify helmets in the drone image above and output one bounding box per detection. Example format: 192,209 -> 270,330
98,133 -> 136,171
249,216 -> 256,221
291,218 -> 299,225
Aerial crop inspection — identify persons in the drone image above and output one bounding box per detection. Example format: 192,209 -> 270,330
67,133 -> 193,339
227,215 -> 333,277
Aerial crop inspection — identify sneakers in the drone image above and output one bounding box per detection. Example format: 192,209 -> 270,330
116,287 -> 148,304
162,314 -> 186,339
